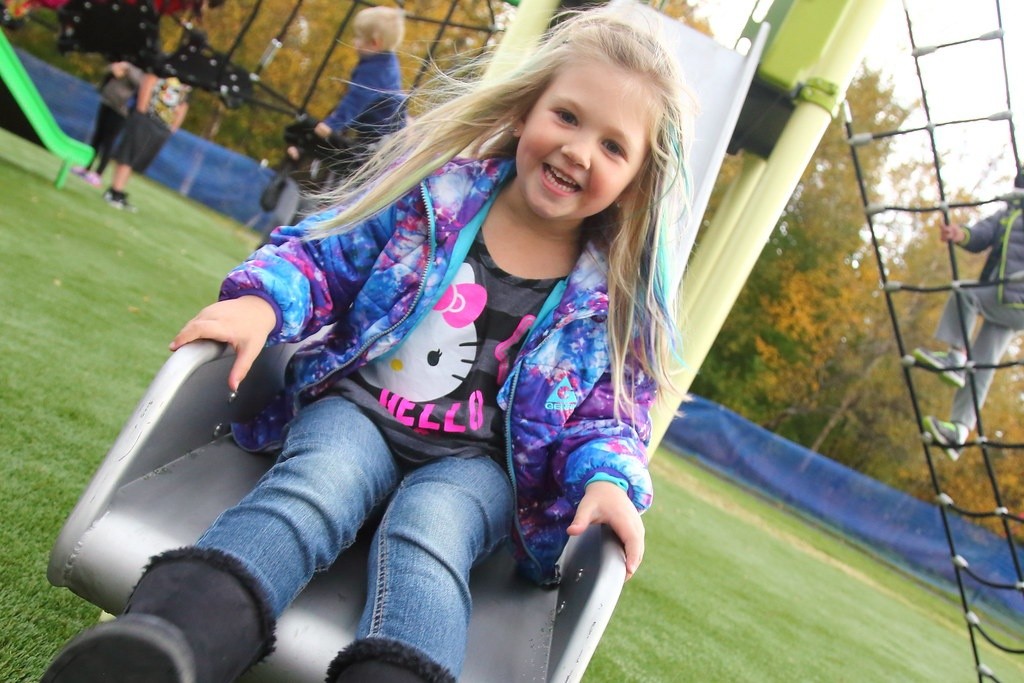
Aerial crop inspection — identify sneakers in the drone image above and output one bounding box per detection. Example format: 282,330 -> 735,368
103,188 -> 138,213
87,172 -> 101,187
923,416 -> 965,461
71,167 -> 88,176
915,345 -> 968,388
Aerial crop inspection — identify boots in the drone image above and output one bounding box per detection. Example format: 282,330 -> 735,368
324,637 -> 457,683
40,547 -> 277,683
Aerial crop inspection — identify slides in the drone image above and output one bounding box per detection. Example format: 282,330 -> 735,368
45,316 -> 649,683
0,27 -> 96,161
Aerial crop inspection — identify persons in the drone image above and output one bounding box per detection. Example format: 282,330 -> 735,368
313,6 -> 407,145
272,145 -> 314,185
912,166 -> 1024,462
37,8 -> 692,683
103,72 -> 194,213
70,60 -> 141,187
